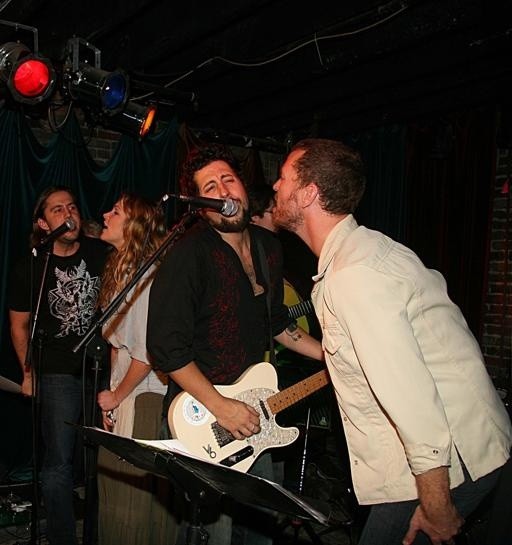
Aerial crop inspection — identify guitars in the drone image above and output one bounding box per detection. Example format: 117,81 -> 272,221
168,359 -> 330,474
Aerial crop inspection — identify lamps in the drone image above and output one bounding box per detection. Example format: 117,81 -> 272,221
0,18 -> 158,138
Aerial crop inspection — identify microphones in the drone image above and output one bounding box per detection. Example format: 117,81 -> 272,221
32,219 -> 76,251
167,192 -> 238,218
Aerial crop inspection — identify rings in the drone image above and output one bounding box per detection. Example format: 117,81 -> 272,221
250,423 -> 257,433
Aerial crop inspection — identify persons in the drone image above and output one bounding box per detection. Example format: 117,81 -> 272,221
96,191 -> 174,442
80,219 -> 102,240
247,180 -> 285,236
267,135 -> 512,545
150,141 -> 324,544
6,185 -> 118,545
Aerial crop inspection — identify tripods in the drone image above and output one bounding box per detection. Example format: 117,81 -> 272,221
278,403 -> 322,545
